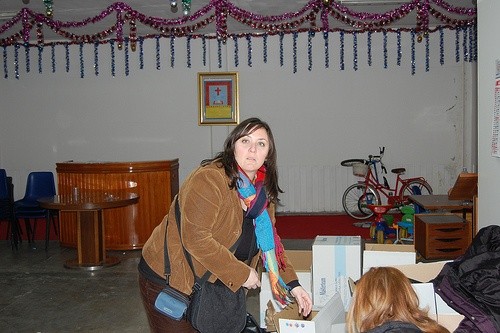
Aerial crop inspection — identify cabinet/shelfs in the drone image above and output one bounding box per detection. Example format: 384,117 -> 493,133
413,212 -> 470,259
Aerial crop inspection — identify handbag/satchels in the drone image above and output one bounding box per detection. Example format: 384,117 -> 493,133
154,287 -> 191,321
188,280 -> 246,333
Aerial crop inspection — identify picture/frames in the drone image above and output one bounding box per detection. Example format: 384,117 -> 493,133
199,71 -> 240,127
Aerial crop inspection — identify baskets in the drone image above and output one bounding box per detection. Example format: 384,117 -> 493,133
352,163 -> 373,177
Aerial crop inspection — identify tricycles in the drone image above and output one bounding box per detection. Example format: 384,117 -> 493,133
361,202 -> 408,244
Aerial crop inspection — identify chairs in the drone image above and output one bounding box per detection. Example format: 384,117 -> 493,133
0,170 -> 58,252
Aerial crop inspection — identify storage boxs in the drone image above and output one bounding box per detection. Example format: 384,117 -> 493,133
312,234 -> 362,312
349,278 -> 437,324
272,293 -> 345,333
259,250 -> 313,328
361,243 -> 416,282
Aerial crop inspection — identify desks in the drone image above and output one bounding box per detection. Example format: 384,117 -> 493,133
38,190 -> 139,269
409,193 -> 474,225
56,157 -> 181,252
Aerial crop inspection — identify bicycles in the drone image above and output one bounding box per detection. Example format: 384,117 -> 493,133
341,147 -> 434,219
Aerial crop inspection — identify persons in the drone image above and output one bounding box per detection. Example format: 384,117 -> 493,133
347,267 -> 451,333
139,118 -> 314,333
